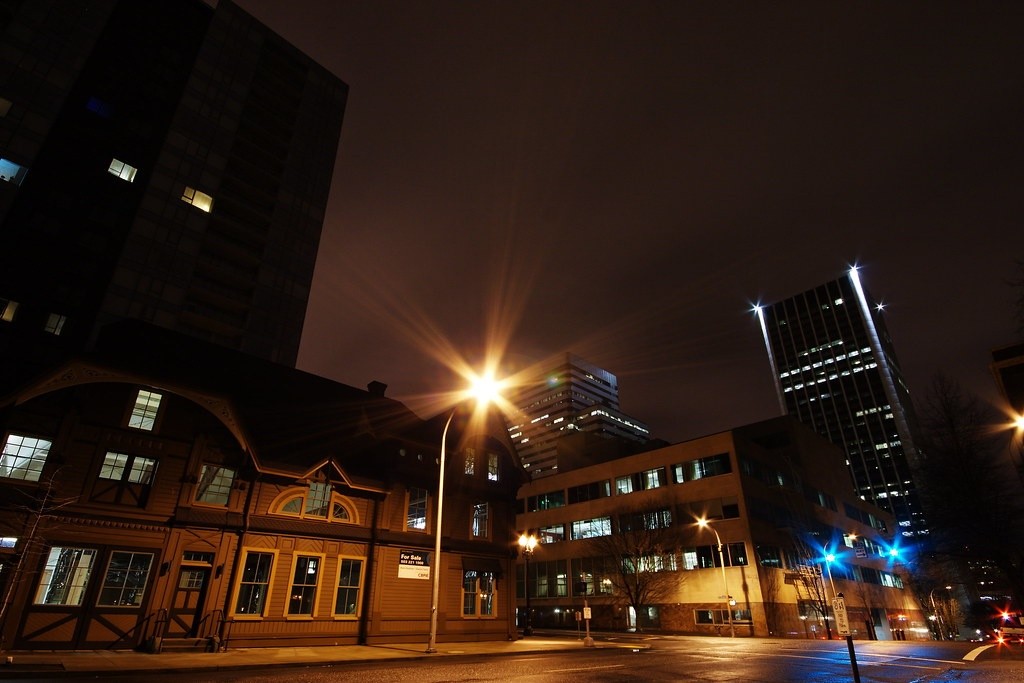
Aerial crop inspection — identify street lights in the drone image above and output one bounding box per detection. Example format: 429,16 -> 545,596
515,531 -> 538,637
929,584 -> 953,641
429,366 -> 509,655
871,548 -> 901,620
823,532 -> 859,639
693,515 -> 734,638
